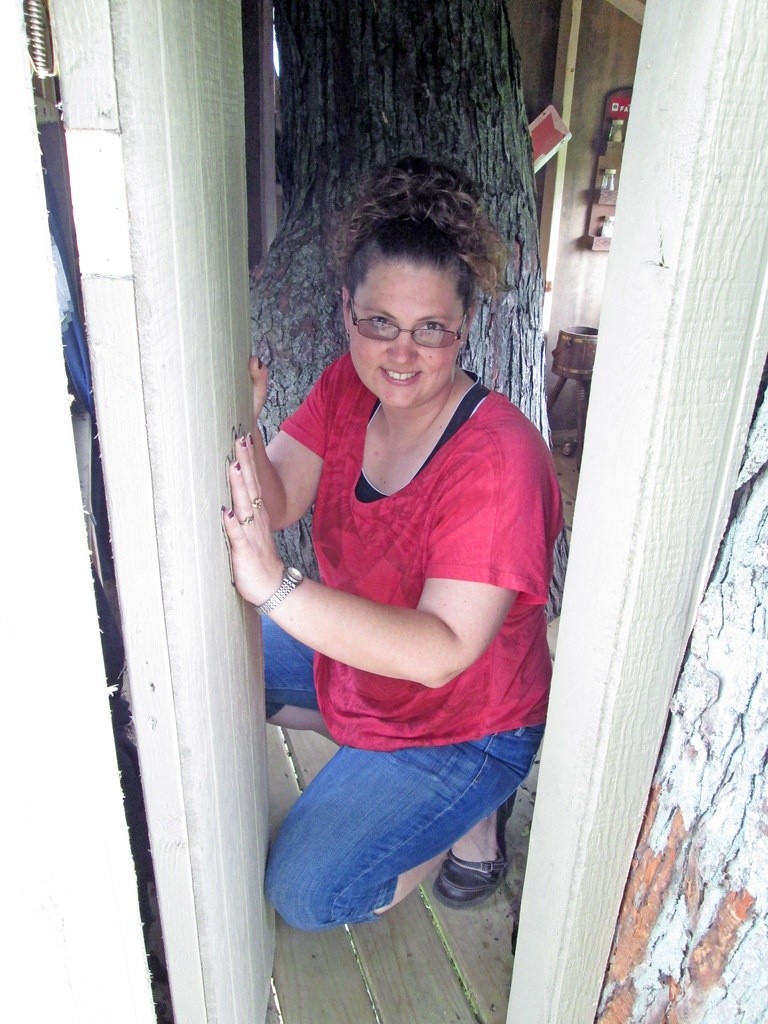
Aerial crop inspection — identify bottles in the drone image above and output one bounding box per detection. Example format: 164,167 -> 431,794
610,120 -> 623,141
602,169 -> 616,190
601,216 -> 615,238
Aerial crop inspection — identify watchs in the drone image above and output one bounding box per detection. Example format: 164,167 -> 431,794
256,566 -> 303,618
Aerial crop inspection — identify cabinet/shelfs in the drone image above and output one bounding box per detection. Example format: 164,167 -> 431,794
584,88 -> 633,251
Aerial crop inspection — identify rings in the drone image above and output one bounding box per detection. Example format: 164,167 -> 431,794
251,497 -> 263,509
240,514 -> 255,526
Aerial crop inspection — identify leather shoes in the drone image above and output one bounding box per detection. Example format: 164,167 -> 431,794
433,788 -> 514,910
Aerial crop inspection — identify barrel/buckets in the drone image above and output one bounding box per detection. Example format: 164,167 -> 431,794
551,326 -> 598,380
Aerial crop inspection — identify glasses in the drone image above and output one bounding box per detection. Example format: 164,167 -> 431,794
348,293 -> 470,349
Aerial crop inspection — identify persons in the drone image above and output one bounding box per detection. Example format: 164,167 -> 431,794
217,156 -> 566,930
